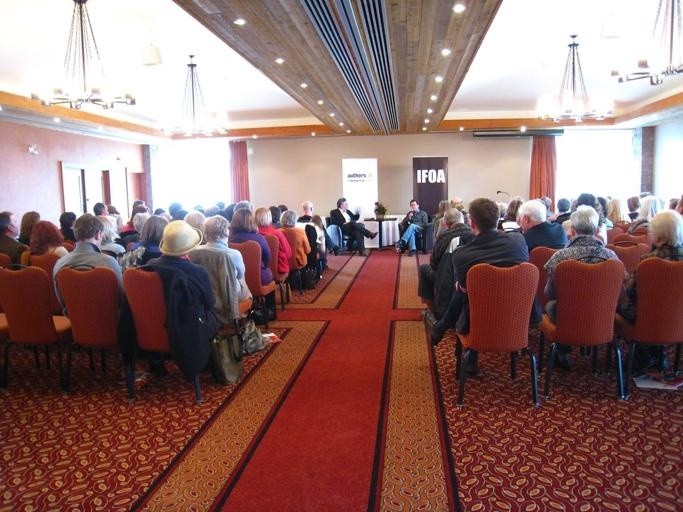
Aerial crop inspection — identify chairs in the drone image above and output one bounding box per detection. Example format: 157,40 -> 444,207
192,245 -> 264,364
0,263 -> 94,389
56,264 -> 130,393
120,264 -> 212,401
282,227 -> 302,297
541,255 -> 627,401
606,257 -> 683,396
398,213 -> 434,254
325,215 -> 365,257
456,261 -> 540,409
229,239 -> 277,322
24,252 -> 67,315
521,247 -> 565,358
438,214 -> 653,272
264,236 -> 289,309
0,222 -> 134,272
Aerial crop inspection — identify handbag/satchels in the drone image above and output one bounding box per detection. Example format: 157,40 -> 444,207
242,319 -> 265,354
211,328 -> 243,384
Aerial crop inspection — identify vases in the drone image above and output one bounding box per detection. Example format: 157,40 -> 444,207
375,213 -> 383,219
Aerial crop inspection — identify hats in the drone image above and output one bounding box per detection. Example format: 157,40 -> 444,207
159,220 -> 203,257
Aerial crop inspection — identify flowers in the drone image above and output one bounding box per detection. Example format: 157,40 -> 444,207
373,200 -> 387,214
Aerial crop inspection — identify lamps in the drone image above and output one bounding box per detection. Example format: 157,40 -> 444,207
28,0 -> 136,120
532,28 -> 613,130
607,0 -> 683,91
158,54 -> 231,140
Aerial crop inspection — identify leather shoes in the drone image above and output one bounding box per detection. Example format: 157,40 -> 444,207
360,251 -> 368,256
422,310 -> 442,345
399,239 -> 405,249
465,351 -> 477,373
370,232 -> 378,238
640,344 -> 669,370
409,250 -> 415,256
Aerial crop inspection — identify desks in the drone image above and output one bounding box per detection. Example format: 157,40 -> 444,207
319,214 -> 406,248
364,217 -> 397,252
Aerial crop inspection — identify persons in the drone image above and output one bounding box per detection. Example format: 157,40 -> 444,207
419,193 -> 683,376
119,220 -> 226,376
1,200 -> 342,331
330,198 -> 378,257
395,200 -> 429,255
